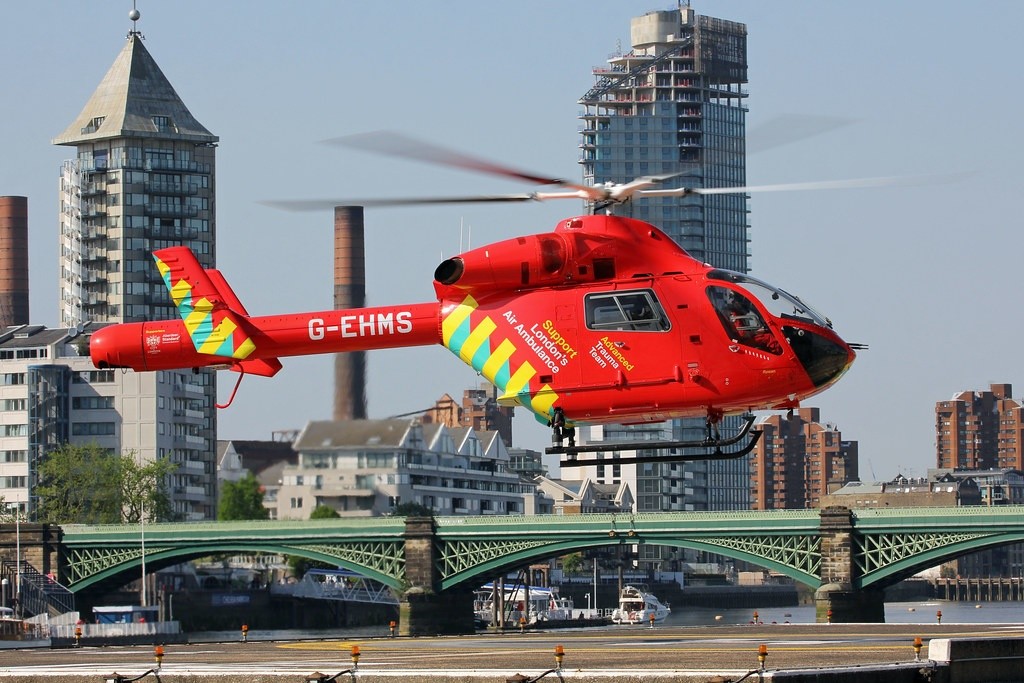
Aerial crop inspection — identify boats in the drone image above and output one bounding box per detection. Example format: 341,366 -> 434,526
473,558 -> 670,630
51,501 -> 189,646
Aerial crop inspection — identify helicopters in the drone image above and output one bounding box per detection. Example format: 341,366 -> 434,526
91,106 -> 895,467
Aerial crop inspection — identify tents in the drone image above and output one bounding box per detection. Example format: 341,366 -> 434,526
503,588 -> 549,613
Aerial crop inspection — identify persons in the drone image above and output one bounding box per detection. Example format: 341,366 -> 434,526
727,295 -> 783,357
